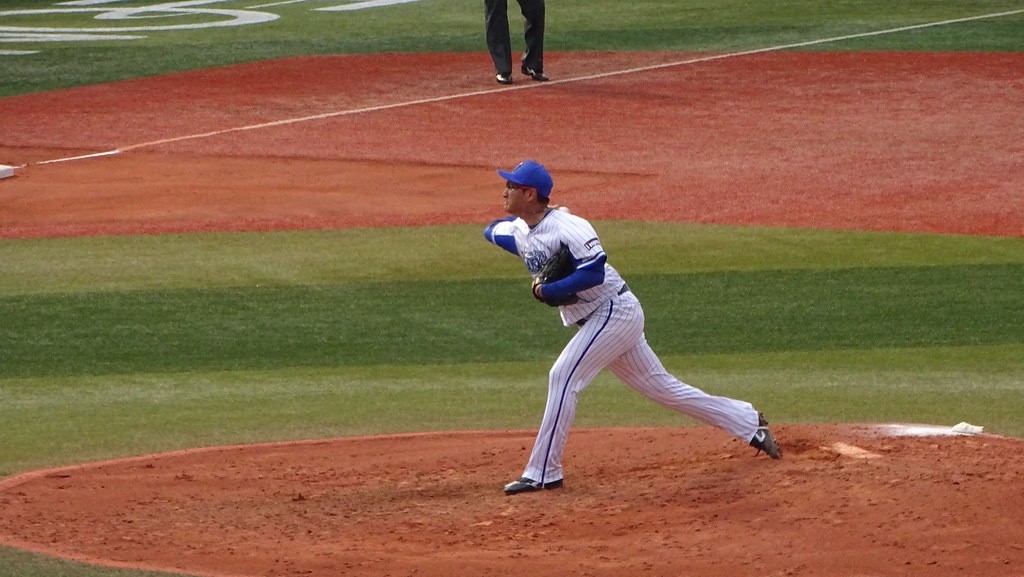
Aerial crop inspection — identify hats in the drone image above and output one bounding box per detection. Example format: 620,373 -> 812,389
497,160 -> 553,198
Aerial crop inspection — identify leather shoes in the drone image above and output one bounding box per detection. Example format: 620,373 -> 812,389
522,65 -> 548,80
496,73 -> 513,84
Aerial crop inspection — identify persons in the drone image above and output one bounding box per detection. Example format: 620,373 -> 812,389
483,0 -> 549,84
482,159 -> 783,496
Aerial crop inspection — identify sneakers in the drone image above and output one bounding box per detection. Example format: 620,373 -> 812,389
504,477 -> 563,493
750,411 -> 781,460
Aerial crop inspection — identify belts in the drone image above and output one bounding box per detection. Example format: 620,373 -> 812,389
576,283 -> 629,326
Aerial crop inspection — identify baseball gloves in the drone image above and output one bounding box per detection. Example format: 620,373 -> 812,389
532,247 -> 579,308
